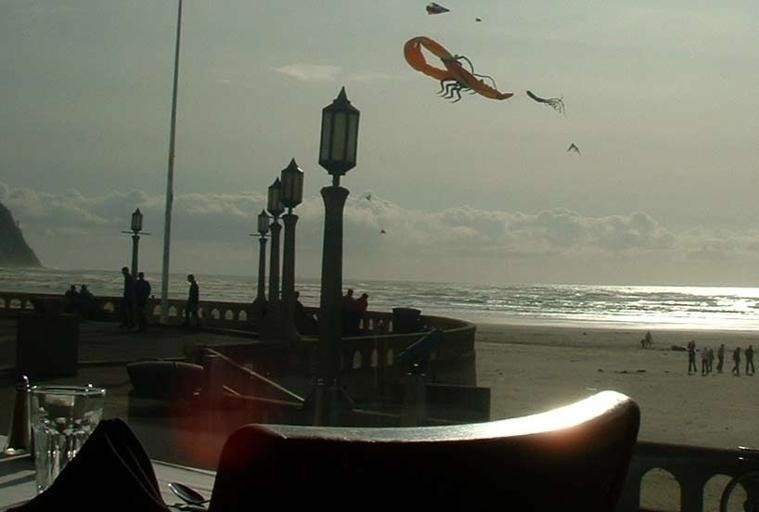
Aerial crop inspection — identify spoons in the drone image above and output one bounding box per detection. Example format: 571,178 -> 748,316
168,479 -> 211,505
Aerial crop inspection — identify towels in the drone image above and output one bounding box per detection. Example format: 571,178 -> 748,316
9,415 -> 167,512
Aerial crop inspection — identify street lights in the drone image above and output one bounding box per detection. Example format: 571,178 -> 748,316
262,175 -> 288,301
130,207 -> 144,277
278,154 -> 305,309
312,76 -> 361,386
253,205 -> 271,303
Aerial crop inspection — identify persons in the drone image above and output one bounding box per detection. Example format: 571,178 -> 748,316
687,341 -> 756,376
120,266 -> 132,330
64,283 -> 81,313
294,290 -> 317,329
135,272 -> 151,309
339,287 -> 368,333
186,273 -> 200,326
79,286 -> 111,316
641,332 -> 654,348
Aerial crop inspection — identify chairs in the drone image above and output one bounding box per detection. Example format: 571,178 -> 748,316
117,359 -> 206,418
207,389 -> 645,512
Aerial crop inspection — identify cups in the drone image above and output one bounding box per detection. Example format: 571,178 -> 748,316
29,384 -> 107,494
